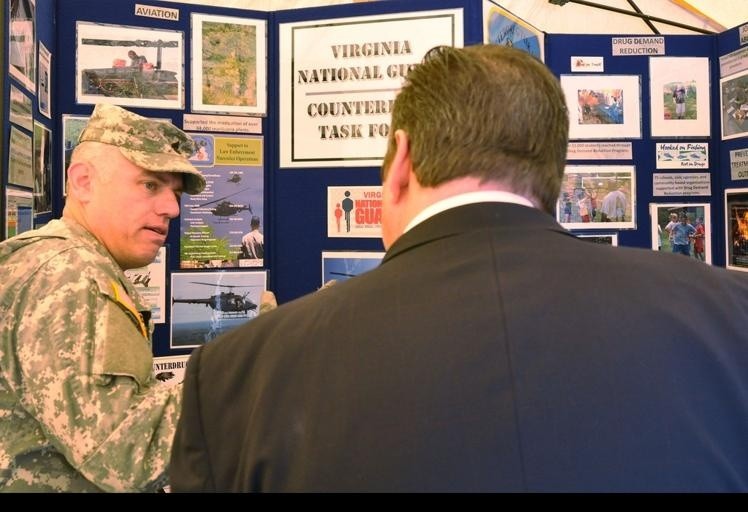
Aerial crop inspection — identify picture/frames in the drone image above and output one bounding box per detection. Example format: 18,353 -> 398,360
321,250 -> 386,288
482,2 -> 546,64
169,268 -> 274,351
554,165 -> 639,229
722,189 -> 748,270
718,70 -> 748,141
277,10 -> 462,172
648,56 -> 710,139
3,1 -> 51,240
179,138 -> 265,267
73,20 -> 185,113
649,203 -> 713,268
558,73 -> 642,139
61,114 -> 90,197
188,11 -> 269,117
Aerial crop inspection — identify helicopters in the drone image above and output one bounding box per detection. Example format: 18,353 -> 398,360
173,282 -> 257,319
227,174 -> 241,183
192,187 -> 253,224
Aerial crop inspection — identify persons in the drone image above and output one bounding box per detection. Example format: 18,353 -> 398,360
562,186 -> 628,223
658,211 -> 704,262
0,102 -> 338,493
673,84 -> 686,119
241,216 -> 263,259
170,44 -> 748,493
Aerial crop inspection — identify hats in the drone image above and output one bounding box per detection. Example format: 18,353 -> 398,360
73,101 -> 206,196
249,216 -> 261,227
